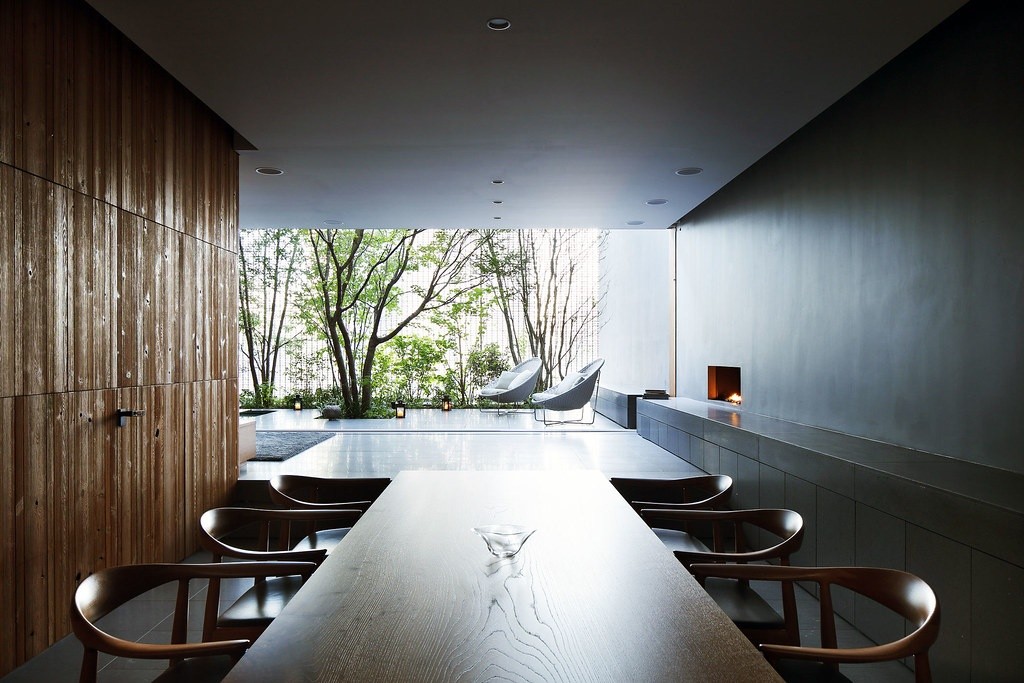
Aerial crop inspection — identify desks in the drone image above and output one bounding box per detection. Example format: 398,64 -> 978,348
219,468 -> 787,683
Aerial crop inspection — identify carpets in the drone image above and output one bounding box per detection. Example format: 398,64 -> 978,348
247,431 -> 335,462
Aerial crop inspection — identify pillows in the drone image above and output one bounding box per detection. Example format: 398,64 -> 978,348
495,370 -> 532,390
557,373 -> 587,394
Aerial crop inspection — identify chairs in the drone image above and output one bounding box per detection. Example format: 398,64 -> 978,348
610,473 -> 942,683
69,474 -> 393,683
532,357 -> 605,426
479,354 -> 544,416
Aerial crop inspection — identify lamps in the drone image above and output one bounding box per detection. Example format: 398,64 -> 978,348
292,395 -> 302,410
395,400 -> 406,418
441,395 -> 452,411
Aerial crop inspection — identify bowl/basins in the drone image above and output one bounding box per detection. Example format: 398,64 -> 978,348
472,525 -> 536,558
